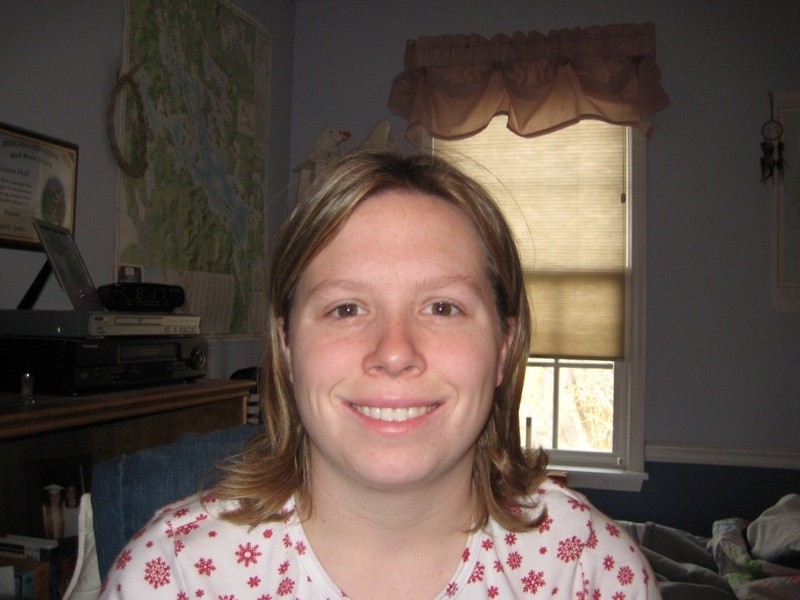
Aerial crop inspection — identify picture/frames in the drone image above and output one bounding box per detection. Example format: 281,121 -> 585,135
33,219 -> 105,312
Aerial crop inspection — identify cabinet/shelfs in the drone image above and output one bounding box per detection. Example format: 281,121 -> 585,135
0,378 -> 257,534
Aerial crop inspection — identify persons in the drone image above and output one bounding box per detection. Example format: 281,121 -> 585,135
96,143 -> 661,600
